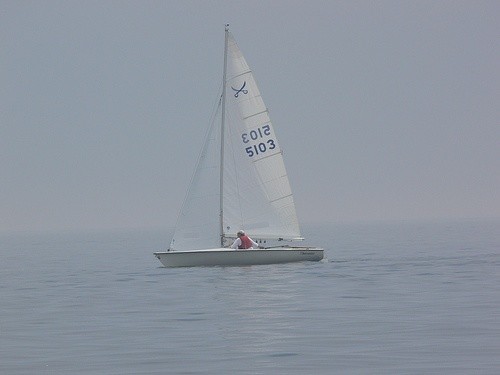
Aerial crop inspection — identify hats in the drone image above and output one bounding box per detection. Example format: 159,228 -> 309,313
237,230 -> 245,236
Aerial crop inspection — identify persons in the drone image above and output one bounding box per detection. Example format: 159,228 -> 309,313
230,230 -> 266,250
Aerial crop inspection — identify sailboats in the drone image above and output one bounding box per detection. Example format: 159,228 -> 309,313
154,23 -> 325,274
